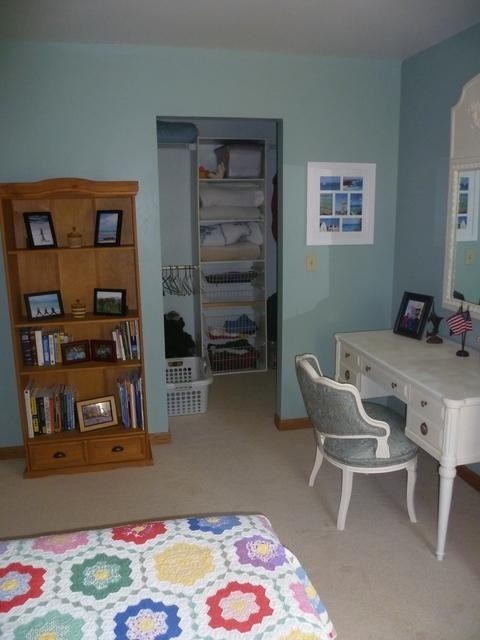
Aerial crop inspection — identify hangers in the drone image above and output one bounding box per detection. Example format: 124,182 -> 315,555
160,264 -> 198,296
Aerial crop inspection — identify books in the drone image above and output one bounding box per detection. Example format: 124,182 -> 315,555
19,321 -> 144,438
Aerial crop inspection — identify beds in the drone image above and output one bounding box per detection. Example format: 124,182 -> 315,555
0,513 -> 338,639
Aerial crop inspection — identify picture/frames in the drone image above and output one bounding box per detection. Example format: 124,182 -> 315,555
60,340 -> 91,364
94,209 -> 121,246
91,339 -> 116,361
393,293 -> 433,340
455,170 -> 477,243
23,288 -> 64,321
22,210 -> 58,248
307,161 -> 377,246
76,395 -> 119,433
94,288 -> 126,318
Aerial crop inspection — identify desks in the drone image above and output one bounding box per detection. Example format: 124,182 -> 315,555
333,328 -> 480,562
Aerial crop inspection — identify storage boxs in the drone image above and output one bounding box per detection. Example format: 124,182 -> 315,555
214,142 -> 261,178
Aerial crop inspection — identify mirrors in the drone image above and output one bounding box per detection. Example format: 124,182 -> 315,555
440,72 -> 479,323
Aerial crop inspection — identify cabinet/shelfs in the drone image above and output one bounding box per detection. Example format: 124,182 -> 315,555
192,136 -> 268,376
0,177 -> 155,479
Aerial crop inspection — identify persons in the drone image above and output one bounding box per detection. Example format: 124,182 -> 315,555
401,302 -> 421,332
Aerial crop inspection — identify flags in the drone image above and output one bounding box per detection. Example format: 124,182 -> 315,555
446,305 -> 464,336
447,310 -> 472,335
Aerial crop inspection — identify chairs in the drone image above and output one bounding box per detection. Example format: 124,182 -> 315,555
293,352 -> 421,531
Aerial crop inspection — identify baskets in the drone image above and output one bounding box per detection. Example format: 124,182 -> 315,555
165,357 -> 214,416
